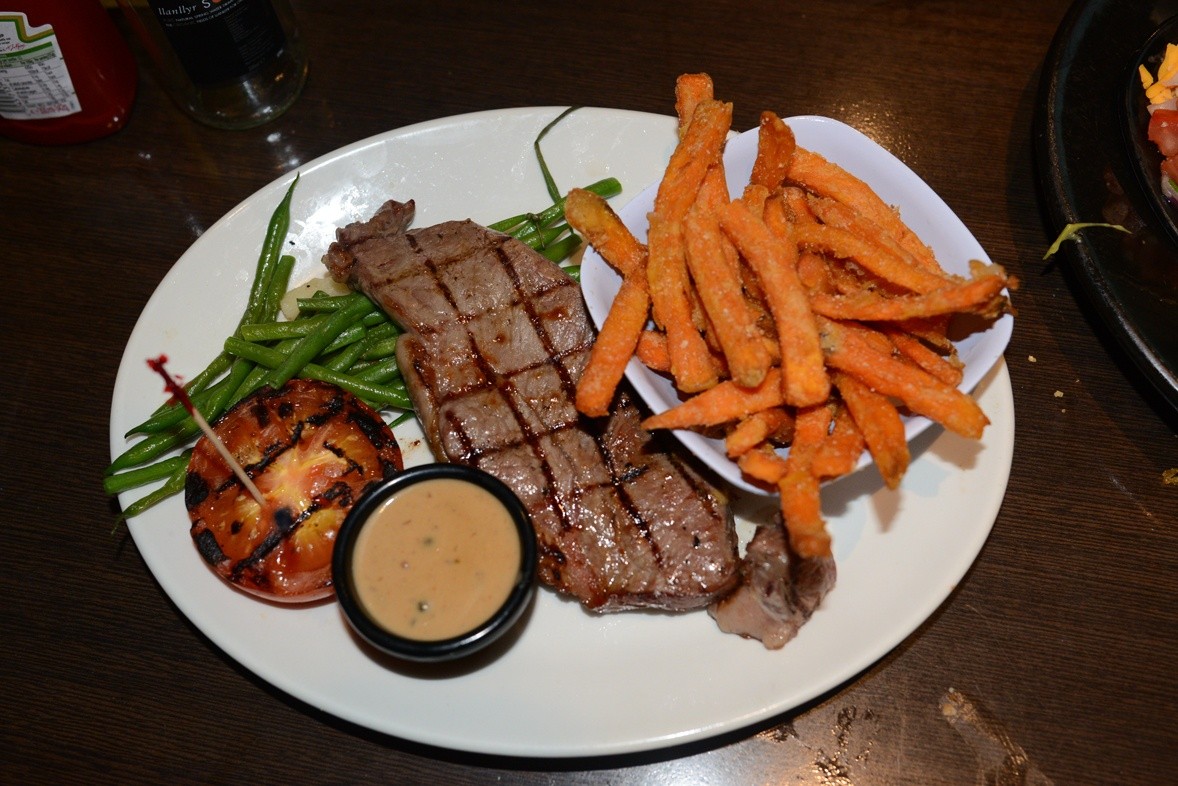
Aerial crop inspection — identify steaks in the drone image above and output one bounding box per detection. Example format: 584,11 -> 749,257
324,198 -> 844,647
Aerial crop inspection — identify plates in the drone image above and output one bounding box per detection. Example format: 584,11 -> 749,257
1018,0 -> 1177,421
581,117 -> 1016,497
105,105 -> 1013,762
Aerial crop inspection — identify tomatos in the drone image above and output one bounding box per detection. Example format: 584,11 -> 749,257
185,379 -> 404,603
1146,109 -> 1178,189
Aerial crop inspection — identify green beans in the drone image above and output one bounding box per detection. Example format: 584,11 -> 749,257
101,169 -> 623,524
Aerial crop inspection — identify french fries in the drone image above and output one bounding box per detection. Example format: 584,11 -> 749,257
562,73 -> 1009,559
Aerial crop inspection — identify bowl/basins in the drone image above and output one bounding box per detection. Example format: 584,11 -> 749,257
329,462 -> 540,667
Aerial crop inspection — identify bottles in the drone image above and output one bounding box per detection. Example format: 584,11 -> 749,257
115,0 -> 309,131
0,0 -> 135,145
